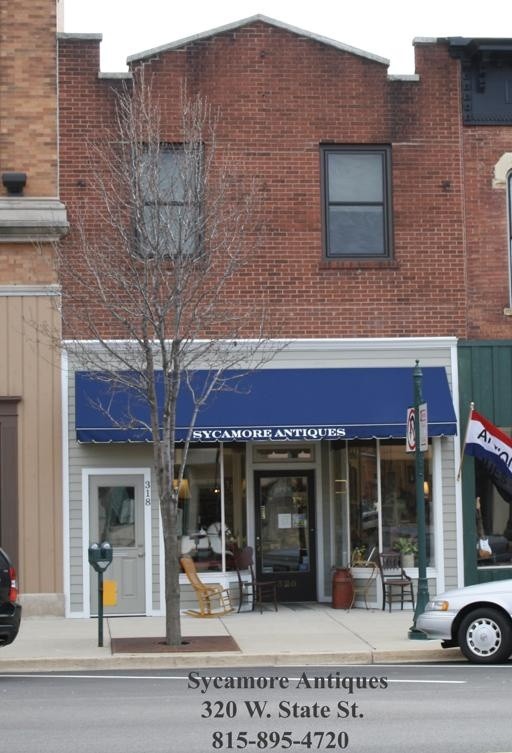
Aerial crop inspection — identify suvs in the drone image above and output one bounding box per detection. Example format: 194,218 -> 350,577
0,548 -> 21,646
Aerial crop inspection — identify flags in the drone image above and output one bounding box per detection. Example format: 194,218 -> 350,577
465,412 -> 512,505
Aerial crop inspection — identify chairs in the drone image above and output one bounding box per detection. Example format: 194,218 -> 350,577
233,551 -> 279,614
346,561 -> 378,613
180,557 -> 237,619
378,552 -> 416,613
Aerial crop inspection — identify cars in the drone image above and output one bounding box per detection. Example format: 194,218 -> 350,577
361,502 -> 379,535
415,578 -> 512,664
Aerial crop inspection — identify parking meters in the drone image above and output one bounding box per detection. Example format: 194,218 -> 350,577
88,542 -> 112,650
